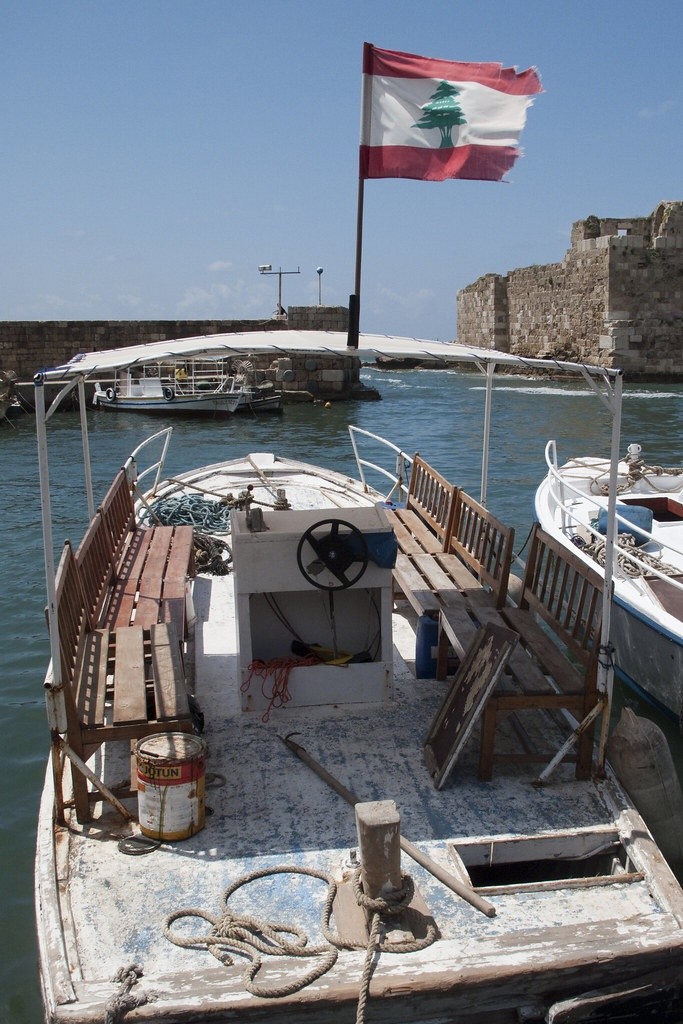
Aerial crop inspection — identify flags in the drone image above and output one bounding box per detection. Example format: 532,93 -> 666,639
359,42 -> 544,181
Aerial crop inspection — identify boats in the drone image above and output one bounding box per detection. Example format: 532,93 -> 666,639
35,329 -> 683,1024
89,353 -> 286,418
0,370 -> 21,422
535,440 -> 683,736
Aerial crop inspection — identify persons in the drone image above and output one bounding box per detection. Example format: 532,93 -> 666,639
176,366 -> 188,387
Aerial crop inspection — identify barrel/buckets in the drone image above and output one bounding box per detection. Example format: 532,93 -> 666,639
130,732 -> 209,841
414,615 -> 447,679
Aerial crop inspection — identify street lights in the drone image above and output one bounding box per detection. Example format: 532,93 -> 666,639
316,267 -> 324,305
259,264 -> 300,314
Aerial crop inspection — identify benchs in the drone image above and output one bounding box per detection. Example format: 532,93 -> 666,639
44,539 -> 194,823
391,486 -> 515,618
436,522 -> 618,780
383,451 -> 458,554
74,466 -> 194,676
